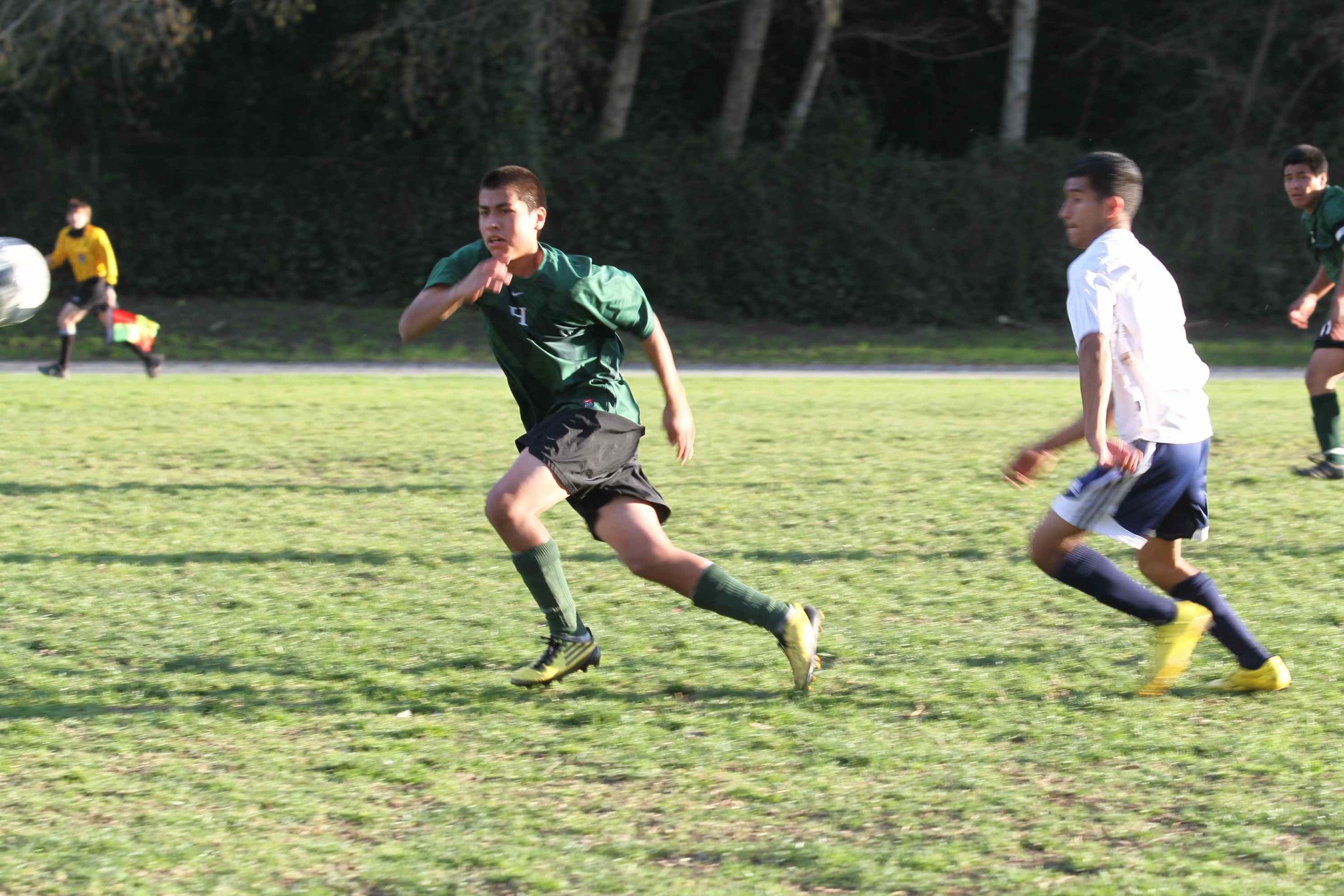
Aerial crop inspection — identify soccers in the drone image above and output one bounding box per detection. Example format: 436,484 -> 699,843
0,235 -> 51,330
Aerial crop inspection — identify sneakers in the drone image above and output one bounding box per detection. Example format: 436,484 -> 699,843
38,366 -> 64,377
148,353 -> 162,379
1221,654 -> 1292,693
1152,597 -> 1214,684
1291,453 -> 1344,481
510,626 -> 599,690
776,602 -> 824,694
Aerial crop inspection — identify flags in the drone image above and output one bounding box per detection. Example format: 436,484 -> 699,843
105,306 -> 161,354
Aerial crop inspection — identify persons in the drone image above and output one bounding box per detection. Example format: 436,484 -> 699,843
36,196 -> 166,382
1282,143 -> 1344,487
397,164 -> 827,702
996,147 -> 1296,702
